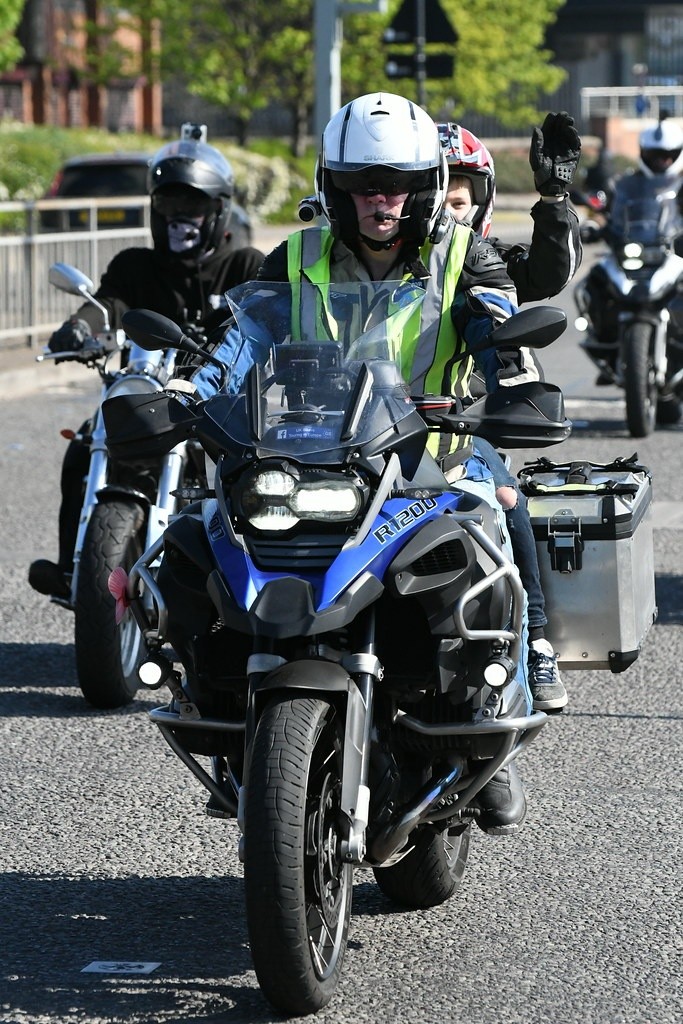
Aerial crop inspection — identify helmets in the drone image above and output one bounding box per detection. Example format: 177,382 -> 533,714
433,123 -> 496,240
149,139 -> 234,258
315,91 -> 449,245
636,121 -> 683,183
599,148 -> 614,160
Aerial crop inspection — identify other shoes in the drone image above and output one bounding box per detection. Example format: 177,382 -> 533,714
594,361 -> 618,387
29,559 -> 73,600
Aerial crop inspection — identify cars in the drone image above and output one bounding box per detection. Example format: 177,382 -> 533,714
37,151 -> 255,250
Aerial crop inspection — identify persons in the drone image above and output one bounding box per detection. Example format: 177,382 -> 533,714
578,119 -> 683,427
28,139 -> 268,649
153,90 -> 587,834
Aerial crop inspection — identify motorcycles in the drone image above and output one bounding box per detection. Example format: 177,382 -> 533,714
35,261 -> 278,711
123,278 -> 660,1016
567,166 -> 683,438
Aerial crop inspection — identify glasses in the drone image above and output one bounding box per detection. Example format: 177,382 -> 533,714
151,193 -> 221,220
330,168 -> 432,196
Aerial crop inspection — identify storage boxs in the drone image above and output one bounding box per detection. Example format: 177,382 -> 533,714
515,459 -> 657,673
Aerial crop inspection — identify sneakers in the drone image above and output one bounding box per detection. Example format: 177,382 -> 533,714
464,759 -> 527,835
527,645 -> 568,711
206,778 -> 239,819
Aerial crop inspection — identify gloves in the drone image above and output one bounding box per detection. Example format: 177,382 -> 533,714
580,221 -> 600,244
48,319 -> 92,365
530,113 -> 582,196
